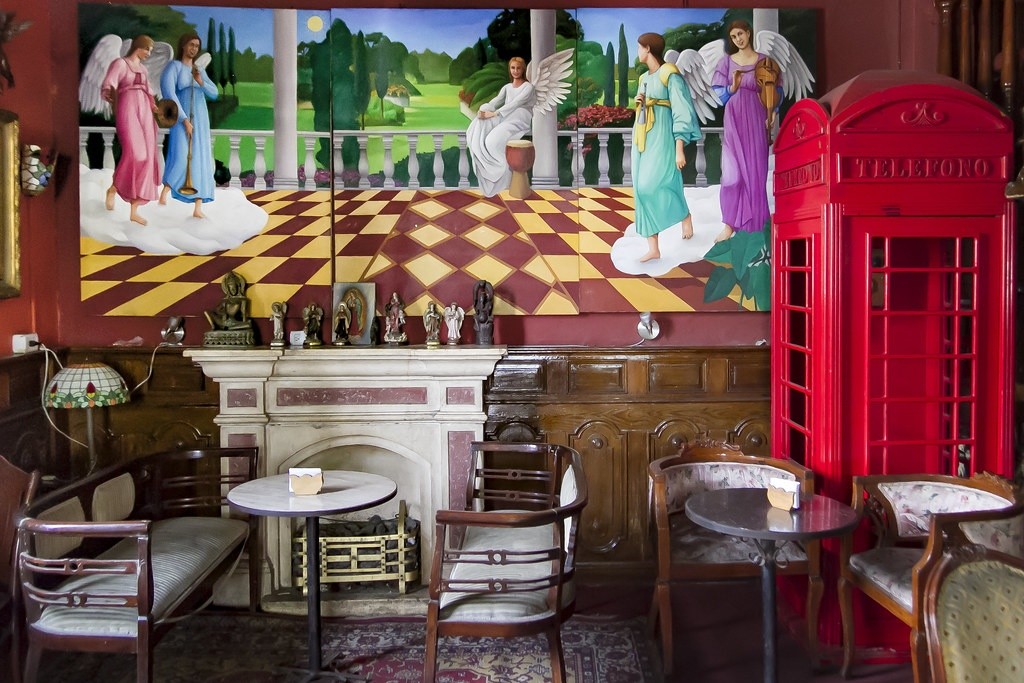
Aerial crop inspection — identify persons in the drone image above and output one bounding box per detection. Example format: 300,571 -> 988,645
472,279 -> 495,331
424,303 -> 443,341
212,271 -> 253,330
304,303 -> 323,341
346,291 -> 360,336
444,301 -> 465,341
269,301 -> 287,341
384,292 -> 406,336
334,302 -> 350,341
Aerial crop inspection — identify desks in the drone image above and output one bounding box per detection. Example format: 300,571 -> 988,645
686,487 -> 858,683
184,345 -> 510,618
226,468 -> 398,683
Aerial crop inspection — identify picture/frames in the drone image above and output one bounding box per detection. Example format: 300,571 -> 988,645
0,108 -> 22,299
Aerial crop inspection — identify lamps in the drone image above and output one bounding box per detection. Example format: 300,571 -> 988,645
19,144 -> 61,196
43,358 -> 130,478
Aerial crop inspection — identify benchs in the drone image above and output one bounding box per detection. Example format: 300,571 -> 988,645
422,439 -> 587,683
13,444 -> 262,683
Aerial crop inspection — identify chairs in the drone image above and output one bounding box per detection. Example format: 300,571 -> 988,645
837,470 -> 1024,683
923,542 -> 1024,683
647,437 -> 825,677
0,454 -> 40,683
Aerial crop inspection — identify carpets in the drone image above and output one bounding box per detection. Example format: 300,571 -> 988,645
34,614 -> 667,683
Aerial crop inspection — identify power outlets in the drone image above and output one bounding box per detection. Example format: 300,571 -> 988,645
13,332 -> 39,352
